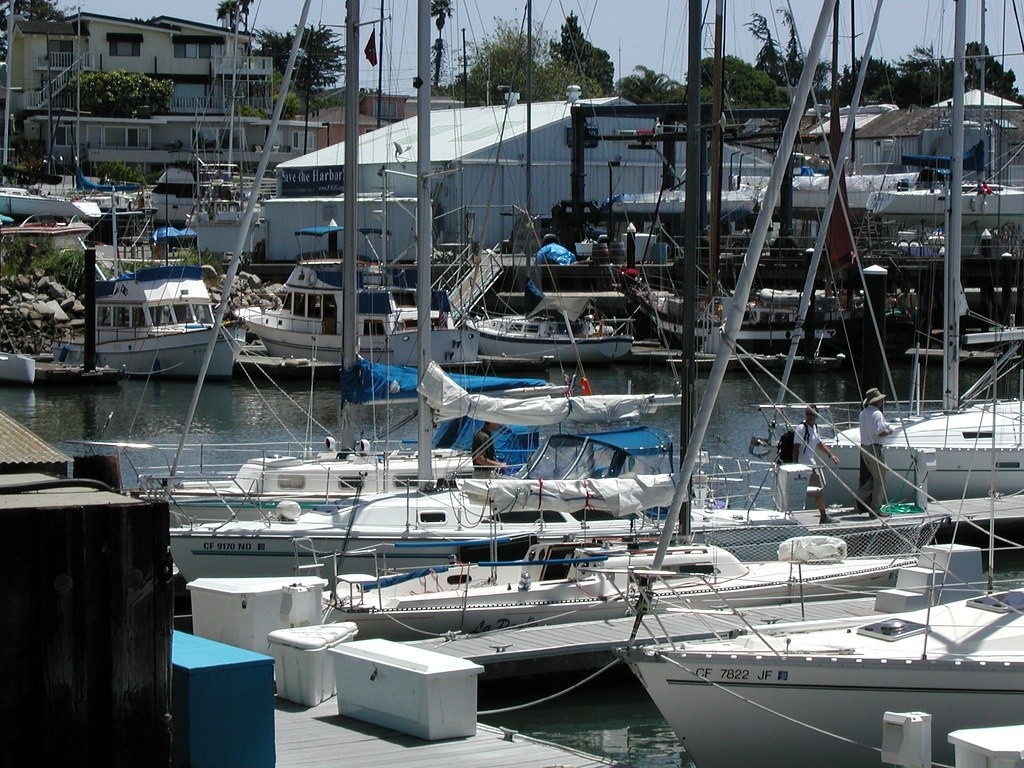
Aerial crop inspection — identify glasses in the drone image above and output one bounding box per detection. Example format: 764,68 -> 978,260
806,412 -> 815,416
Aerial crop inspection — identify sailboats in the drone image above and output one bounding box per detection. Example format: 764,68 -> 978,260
124,0 -> 1024,768
141,12 -> 255,231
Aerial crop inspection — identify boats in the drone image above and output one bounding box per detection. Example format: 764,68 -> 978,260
41,184 -> 238,386
231,221 -> 484,381
0,185 -> 157,247
465,284 -> 634,364
632,279 -> 839,354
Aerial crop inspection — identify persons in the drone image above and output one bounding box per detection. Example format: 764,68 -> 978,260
857,388 -> 893,519
793,405 -> 839,524
473,421 -> 508,478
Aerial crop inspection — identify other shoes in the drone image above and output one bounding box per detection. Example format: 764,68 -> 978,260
870,512 -> 892,520
820,515 -> 840,523
855,499 -> 863,514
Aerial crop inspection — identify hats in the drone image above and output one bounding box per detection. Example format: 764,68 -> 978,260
863,388 -> 886,405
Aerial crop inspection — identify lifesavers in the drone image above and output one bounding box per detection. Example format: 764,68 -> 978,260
731,241 -> 744,255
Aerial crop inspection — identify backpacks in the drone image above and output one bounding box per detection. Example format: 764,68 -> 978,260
777,422 -> 808,463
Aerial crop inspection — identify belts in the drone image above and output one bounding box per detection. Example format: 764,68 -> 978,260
871,444 -> 883,446
475,468 -> 493,471
